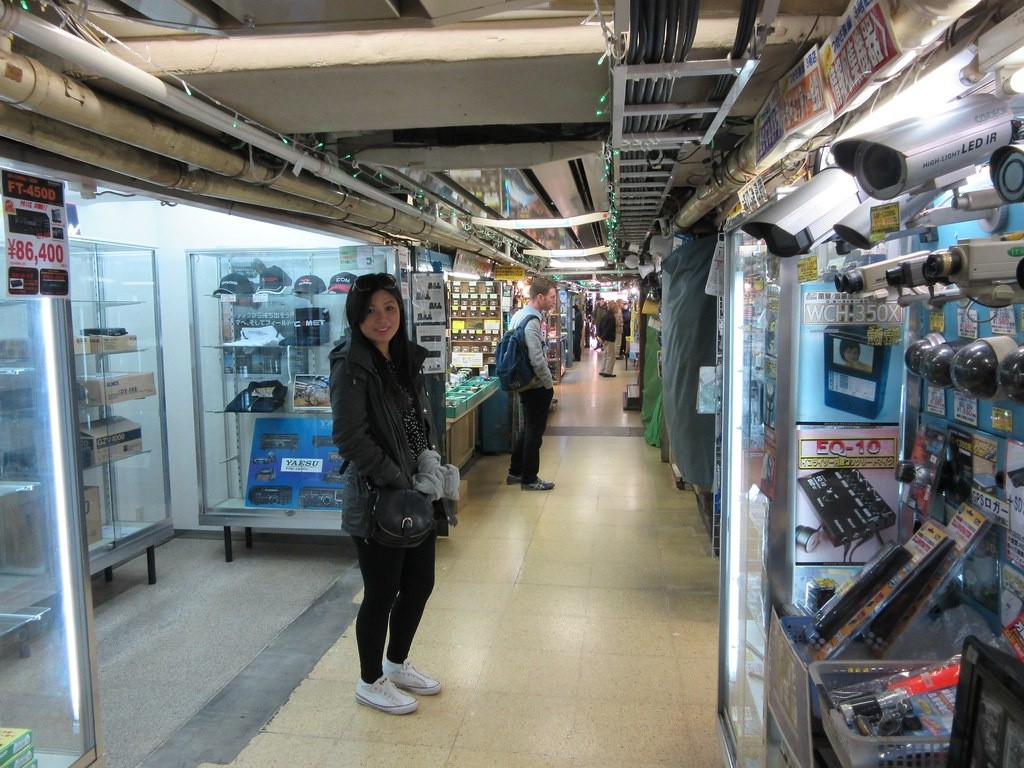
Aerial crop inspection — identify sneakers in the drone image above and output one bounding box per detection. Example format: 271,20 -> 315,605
521,477 -> 555,490
355,676 -> 418,715
506,474 -> 524,484
383,658 -> 441,696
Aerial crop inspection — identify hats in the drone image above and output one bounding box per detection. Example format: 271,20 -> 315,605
292,275 -> 326,294
617,299 -> 626,304
328,272 -> 357,292
256,264 -> 291,294
213,273 -> 253,295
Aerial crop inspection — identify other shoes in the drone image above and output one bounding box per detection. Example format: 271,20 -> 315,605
594,344 -> 601,350
599,373 -> 603,375
603,374 -> 616,377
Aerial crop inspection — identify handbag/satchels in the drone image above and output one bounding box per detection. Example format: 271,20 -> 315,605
368,489 -> 434,547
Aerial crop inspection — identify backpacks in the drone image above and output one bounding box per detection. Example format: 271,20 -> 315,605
495,314 -> 541,391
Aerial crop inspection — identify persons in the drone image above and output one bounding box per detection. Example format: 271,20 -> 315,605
592,298 -> 631,377
573,305 -> 582,361
840,340 -> 872,371
507,278 -> 557,490
329,273 -> 441,713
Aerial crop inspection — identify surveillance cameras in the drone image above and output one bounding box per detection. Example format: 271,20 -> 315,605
742,92 -> 1024,405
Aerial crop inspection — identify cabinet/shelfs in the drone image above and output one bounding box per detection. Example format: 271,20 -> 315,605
188,247 -> 400,536
0,235 -> 176,615
443,407 -> 480,473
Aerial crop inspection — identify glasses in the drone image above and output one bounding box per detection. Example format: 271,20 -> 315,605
351,273 -> 397,292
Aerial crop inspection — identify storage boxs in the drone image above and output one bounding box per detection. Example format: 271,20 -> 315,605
1,446 -> 41,480
2,387 -> 34,412
453,480 -> 468,514
0,486 -> 103,568
443,377 -> 500,417
2,336 -> 89,358
89,333 -> 138,352
0,728 -> 38,768
78,417 -> 142,467
75,370 -> 157,407
